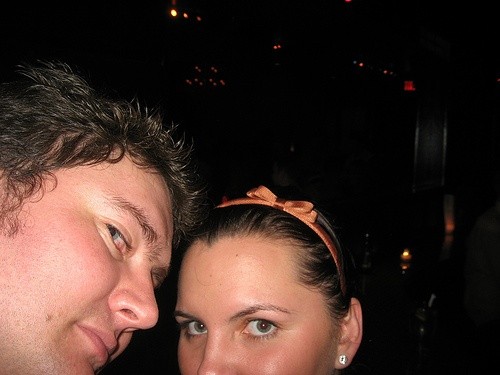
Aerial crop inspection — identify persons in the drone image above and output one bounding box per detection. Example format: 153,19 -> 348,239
1,46 -> 218,375
169,185 -> 365,375
